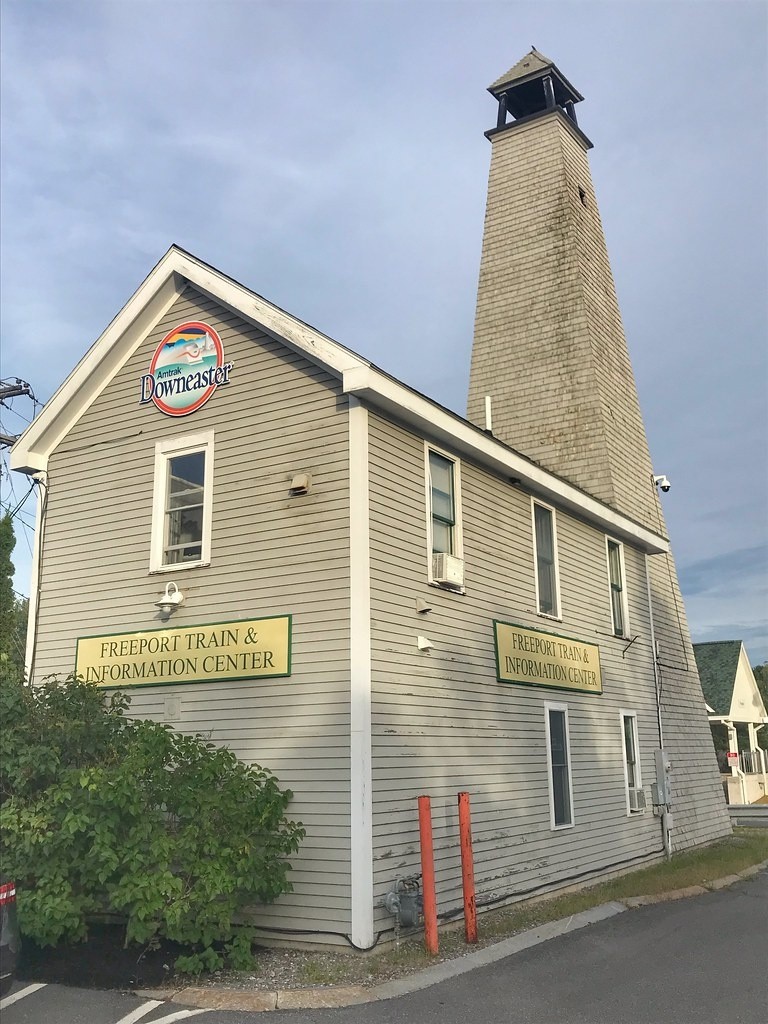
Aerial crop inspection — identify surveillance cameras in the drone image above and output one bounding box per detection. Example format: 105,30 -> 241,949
660,479 -> 671,492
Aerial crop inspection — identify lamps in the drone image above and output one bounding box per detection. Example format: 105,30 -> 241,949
154,582 -> 184,613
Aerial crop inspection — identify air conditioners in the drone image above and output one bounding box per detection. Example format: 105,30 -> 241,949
432,553 -> 465,587
629,788 -> 647,811
